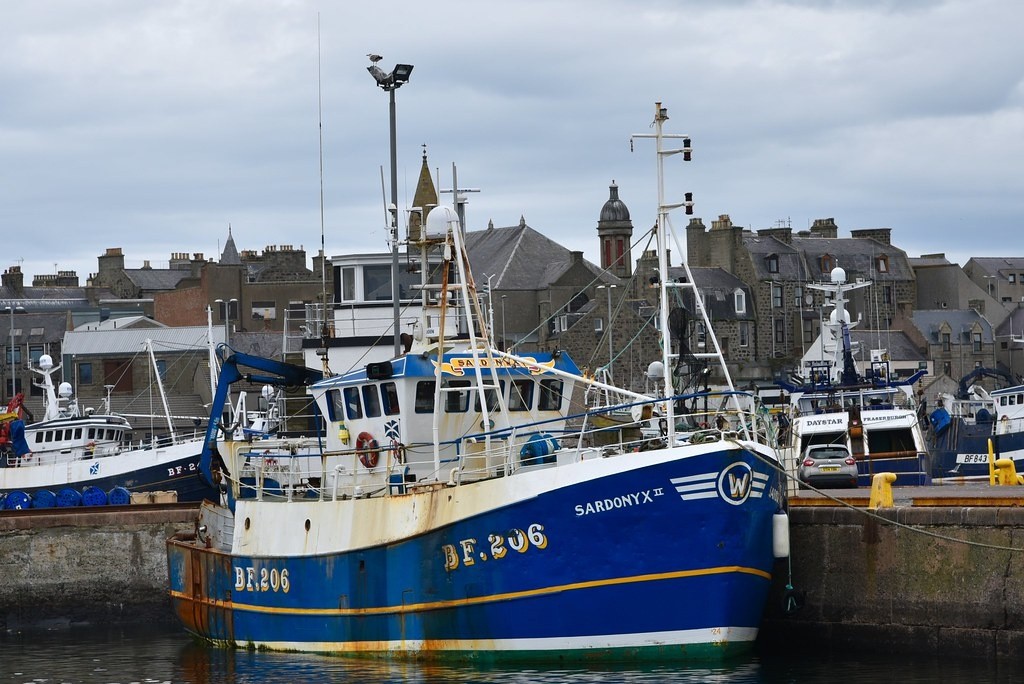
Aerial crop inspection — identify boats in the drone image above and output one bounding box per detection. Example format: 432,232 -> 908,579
0,51 -> 1023,664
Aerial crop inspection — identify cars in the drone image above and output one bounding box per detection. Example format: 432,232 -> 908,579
793,444 -> 859,488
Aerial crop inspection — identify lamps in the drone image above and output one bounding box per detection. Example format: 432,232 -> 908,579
421,351 -> 429,359
553,349 -> 561,357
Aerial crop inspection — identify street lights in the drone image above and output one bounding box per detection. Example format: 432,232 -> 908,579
214,298 -> 239,426
3,301 -> 25,398
597,282 -> 618,384
501,293 -> 507,353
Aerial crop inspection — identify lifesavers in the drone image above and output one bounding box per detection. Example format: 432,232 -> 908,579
355,431 -> 378,468
1001,415 -> 1009,422
264,450 -> 273,462
86,442 -> 96,447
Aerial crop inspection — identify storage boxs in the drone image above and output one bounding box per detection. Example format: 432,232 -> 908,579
130,490 -> 178,504
